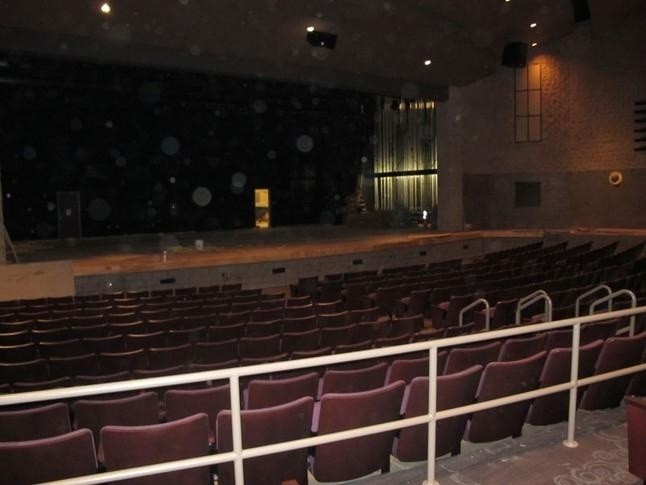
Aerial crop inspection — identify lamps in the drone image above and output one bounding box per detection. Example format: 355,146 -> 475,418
501,1 -> 529,68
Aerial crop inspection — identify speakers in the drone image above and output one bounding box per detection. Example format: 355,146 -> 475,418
502,42 -> 526,66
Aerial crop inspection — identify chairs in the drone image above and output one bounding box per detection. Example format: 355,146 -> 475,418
1,240 -> 645,484
